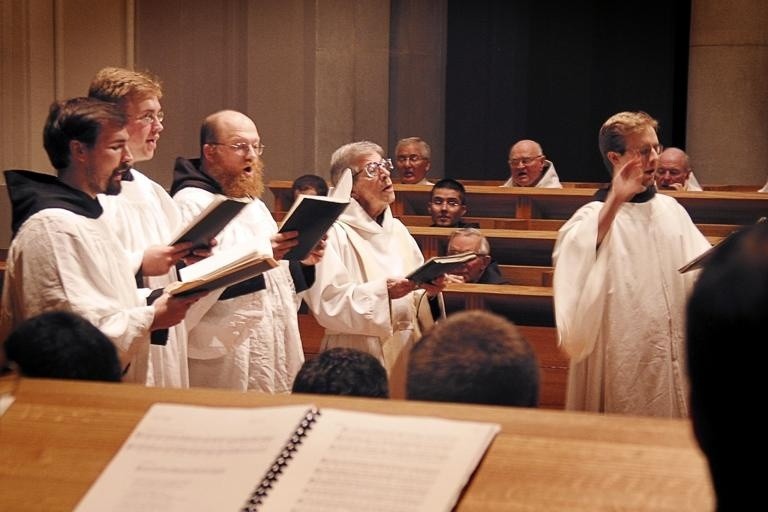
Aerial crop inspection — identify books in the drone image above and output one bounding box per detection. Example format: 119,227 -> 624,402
405,251 -> 478,285
276,168 -> 353,264
168,251 -> 281,301
167,193 -> 250,259
72,400 -> 503,512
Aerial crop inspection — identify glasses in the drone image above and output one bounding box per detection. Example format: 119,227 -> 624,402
397,156 -> 426,162
353,158 -> 395,179
431,200 -> 462,207
508,156 -> 542,168
623,145 -> 662,159
203,140 -> 265,157
122,111 -> 165,124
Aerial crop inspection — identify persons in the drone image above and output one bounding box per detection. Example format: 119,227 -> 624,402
0,97 -> 210,385
404,310 -> 539,409
683,224 -> 767,512
169,109 -> 328,396
292,345 -> 390,399
0,310 -> 122,383
552,111 -> 713,416
88,65 -> 217,387
395,137 -> 436,187
498,139 -> 563,188
426,177 -> 479,228
654,146 -> 703,191
292,174 -> 327,202
447,231 -> 514,284
302,141 -> 448,398
